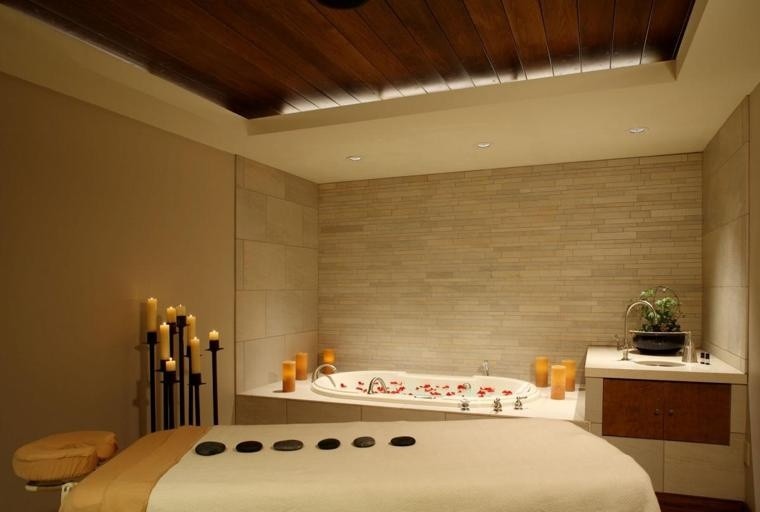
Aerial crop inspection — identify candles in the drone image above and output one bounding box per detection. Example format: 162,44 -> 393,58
535,357 -> 576,400
281,348 -> 335,392
146,297 -> 218,374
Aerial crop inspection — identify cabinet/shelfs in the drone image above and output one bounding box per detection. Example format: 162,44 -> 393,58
584,377 -> 747,504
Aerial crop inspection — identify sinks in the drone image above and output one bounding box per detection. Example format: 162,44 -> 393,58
636,359 -> 686,368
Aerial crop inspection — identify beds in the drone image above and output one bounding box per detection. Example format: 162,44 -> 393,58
10,415 -> 661,512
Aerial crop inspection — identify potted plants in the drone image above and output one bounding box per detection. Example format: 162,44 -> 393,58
628,287 -> 689,357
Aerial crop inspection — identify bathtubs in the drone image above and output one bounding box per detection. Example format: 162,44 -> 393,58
310,369 -> 540,408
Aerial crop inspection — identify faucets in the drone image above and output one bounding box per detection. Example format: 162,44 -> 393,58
311,364 -> 336,381
620,299 -> 660,361
